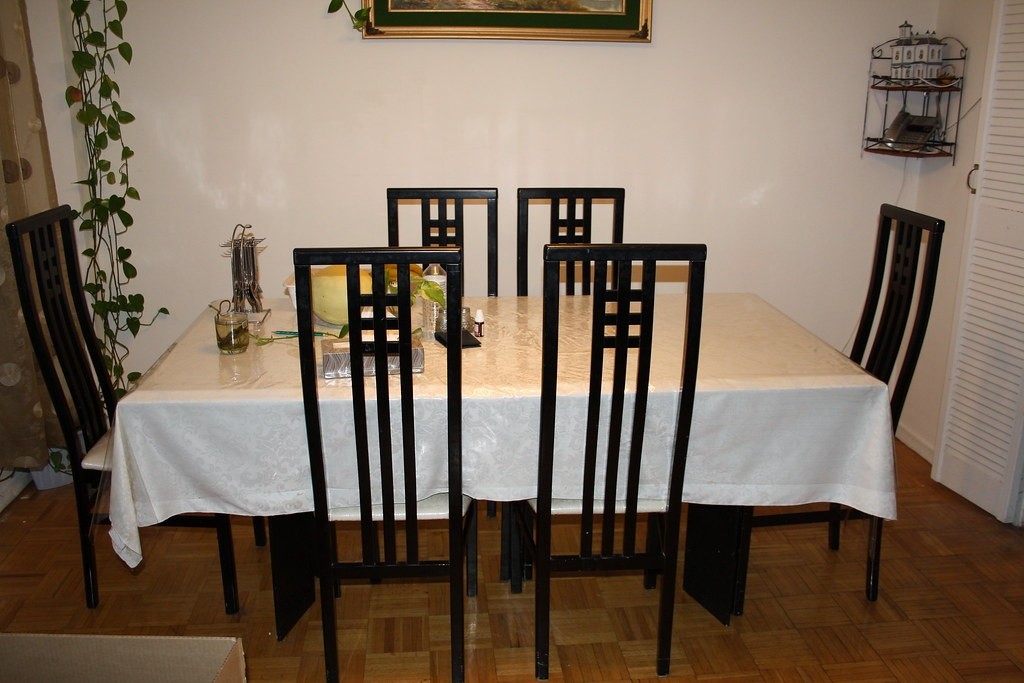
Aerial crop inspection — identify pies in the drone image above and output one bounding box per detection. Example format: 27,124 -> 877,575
310,263 -> 423,326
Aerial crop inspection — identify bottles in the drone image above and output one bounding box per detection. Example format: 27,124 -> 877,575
423,261 -> 447,330
474,310 -> 484,337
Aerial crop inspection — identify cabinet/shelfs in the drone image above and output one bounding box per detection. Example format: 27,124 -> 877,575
860,20 -> 968,167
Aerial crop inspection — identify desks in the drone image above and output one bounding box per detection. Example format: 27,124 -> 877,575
107,288 -> 900,641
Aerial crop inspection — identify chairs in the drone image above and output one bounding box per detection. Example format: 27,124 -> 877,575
387,187 -> 513,582
509,243 -> 707,681
294,247 -> 481,683
515,187 -> 634,580
4,204 -> 268,616
734,204 -> 947,616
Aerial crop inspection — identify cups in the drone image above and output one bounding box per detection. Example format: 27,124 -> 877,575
214,312 -> 250,354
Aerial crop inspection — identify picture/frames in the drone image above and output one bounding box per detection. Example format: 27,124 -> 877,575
361,0 -> 653,44
